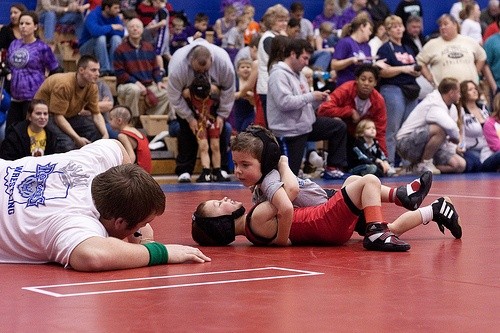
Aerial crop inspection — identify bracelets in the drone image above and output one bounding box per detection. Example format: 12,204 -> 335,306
141,241 -> 168,265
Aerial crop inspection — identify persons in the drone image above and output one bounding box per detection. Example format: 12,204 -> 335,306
232,123 -> 462,246
191,155 -> 433,251
0,0 -> 500,183
0,138 -> 211,270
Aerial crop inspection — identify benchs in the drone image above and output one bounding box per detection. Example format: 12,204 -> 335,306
36,22 -> 169,138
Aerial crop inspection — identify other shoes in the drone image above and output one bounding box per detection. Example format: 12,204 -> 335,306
398,164 -> 414,174
195,168 -> 212,183
131,115 -> 143,129
415,159 -> 441,176
211,168 -> 231,182
177,172 -> 191,183
324,170 -> 352,180
431,197 -> 462,239
362,222 -> 411,252
395,170 -> 433,211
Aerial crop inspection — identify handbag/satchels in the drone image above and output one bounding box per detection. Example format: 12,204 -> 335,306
401,84 -> 420,101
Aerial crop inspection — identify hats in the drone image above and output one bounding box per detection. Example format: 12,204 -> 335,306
191,77 -> 210,99
192,215 -> 236,247
